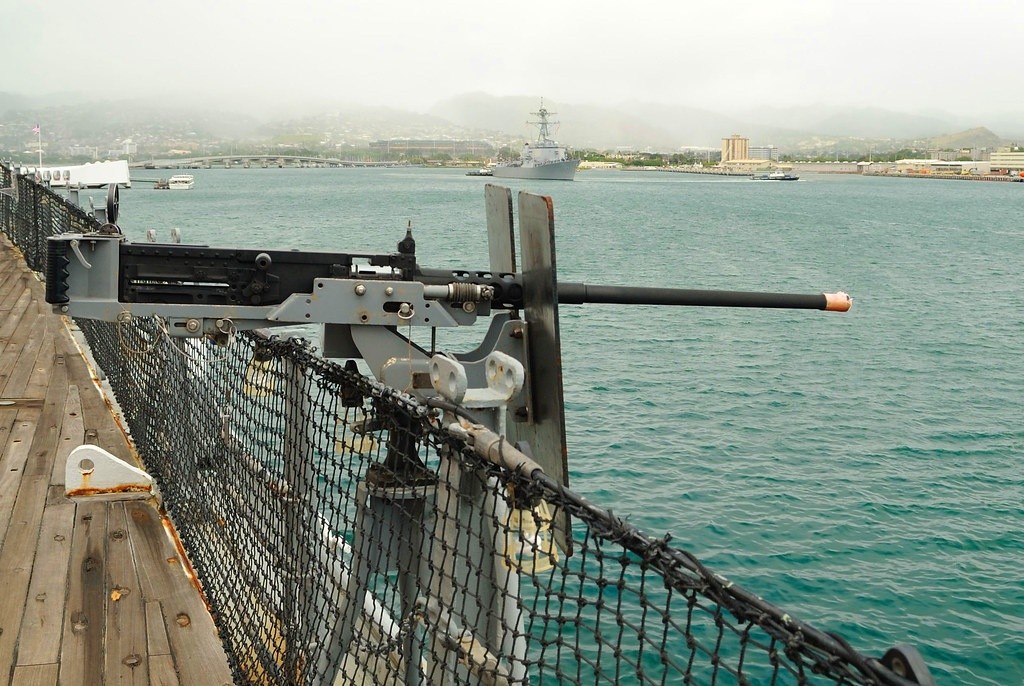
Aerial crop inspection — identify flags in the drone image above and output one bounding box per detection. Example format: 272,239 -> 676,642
33,124 -> 39,135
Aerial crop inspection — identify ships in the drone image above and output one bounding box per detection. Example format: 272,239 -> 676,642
489,96 -> 581,181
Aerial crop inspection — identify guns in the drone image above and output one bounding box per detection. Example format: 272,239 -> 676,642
44,181 -> 855,363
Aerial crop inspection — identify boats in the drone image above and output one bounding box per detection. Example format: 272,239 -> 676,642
749,168 -> 802,181
168,174 -> 196,191
